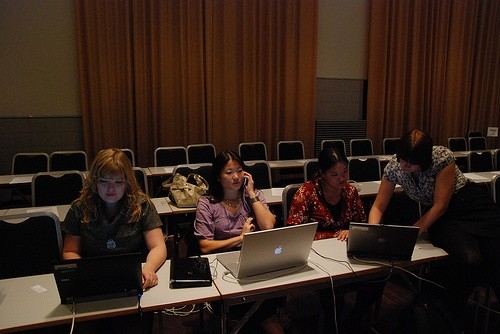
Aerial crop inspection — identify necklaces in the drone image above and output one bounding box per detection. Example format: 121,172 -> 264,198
105,237 -> 117,251
225,194 -> 243,209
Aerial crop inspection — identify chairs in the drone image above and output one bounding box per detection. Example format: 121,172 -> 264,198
0,130 -> 500,334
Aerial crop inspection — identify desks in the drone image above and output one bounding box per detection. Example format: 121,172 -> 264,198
453,149 -> 495,161
270,157 -> 318,171
200,230 -> 450,334
0,169 -> 152,204
149,160 -> 269,200
0,198 -> 173,258
462,170 -> 500,202
351,181 -> 404,207
0,256 -> 221,334
164,188 -> 286,256
344,154 -> 393,162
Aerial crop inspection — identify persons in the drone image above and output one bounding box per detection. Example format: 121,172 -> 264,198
366,128 -> 500,306
61,149 -> 167,334
286,148 -> 387,334
194,150 -> 284,334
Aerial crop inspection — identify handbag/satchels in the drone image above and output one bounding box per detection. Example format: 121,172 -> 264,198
161,167 -> 211,208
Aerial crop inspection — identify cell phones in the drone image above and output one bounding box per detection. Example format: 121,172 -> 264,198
243,177 -> 247,185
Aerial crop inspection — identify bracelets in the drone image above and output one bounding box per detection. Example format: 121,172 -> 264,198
250,196 -> 260,204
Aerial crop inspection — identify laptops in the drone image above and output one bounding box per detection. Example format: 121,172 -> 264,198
168,256 -> 212,289
346,222 -> 420,262
50,252 -> 143,304
215,221 -> 319,283
